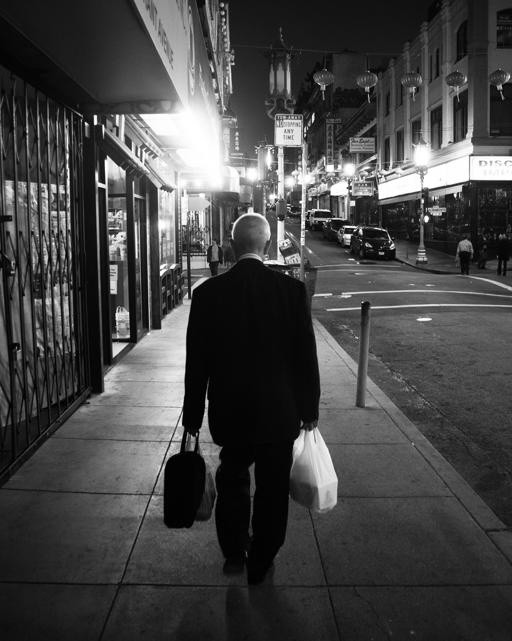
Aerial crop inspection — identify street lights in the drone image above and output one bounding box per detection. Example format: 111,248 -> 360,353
343,163 -> 355,219
256,28 -> 297,261
410,134 -> 429,264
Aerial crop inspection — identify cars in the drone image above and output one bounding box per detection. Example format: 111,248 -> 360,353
285,203 -> 301,218
306,209 -> 395,259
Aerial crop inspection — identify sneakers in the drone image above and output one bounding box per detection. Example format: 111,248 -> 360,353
247,560 -> 273,585
223,551 -> 246,576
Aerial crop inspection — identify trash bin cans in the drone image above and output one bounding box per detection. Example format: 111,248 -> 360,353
267,264 -> 318,308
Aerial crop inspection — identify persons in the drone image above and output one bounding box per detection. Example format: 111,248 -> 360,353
207,238 -> 223,276
180,212 -> 322,586
455,231 -> 512,277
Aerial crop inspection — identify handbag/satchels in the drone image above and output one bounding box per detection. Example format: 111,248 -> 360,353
164,453 -> 205,529
